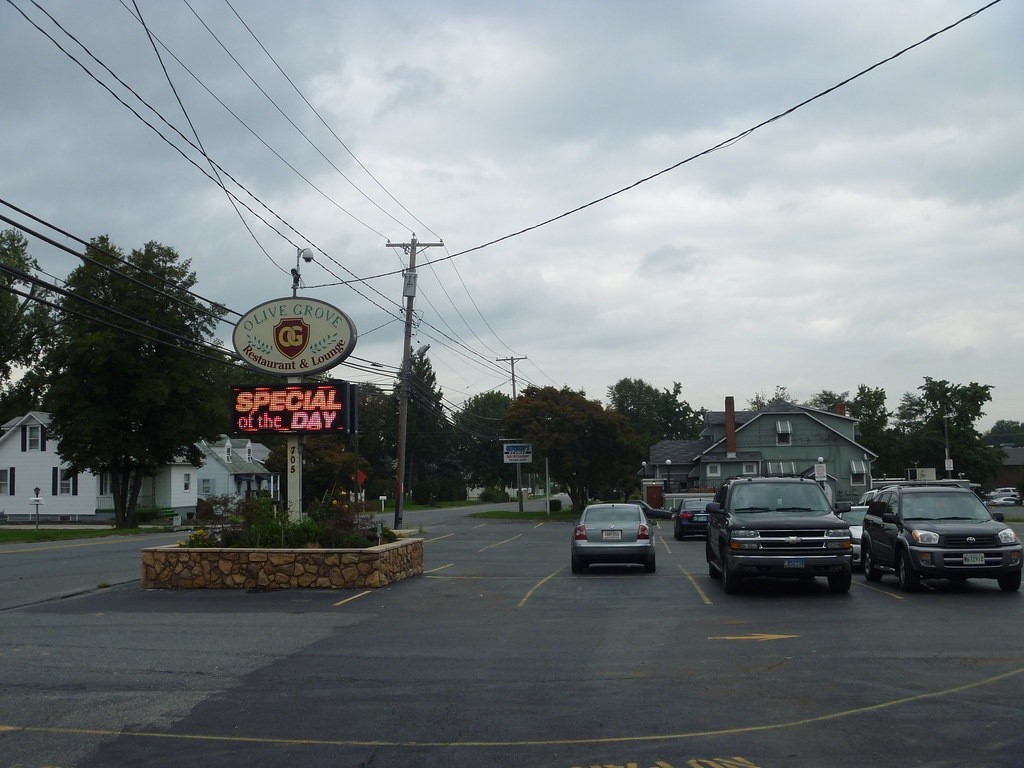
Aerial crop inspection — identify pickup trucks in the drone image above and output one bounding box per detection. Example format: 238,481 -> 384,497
626,500 -> 674,519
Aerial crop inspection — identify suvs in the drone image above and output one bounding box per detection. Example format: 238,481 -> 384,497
706,473 -> 853,594
861,483 -> 1023,592
986,488 -> 1020,498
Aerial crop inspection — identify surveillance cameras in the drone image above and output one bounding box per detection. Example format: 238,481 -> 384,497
303,249 -> 313,263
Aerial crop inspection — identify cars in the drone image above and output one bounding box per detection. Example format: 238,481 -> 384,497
674,498 -> 713,541
838,506 -> 871,573
989,497 -> 1024,505
571,503 -> 658,574
